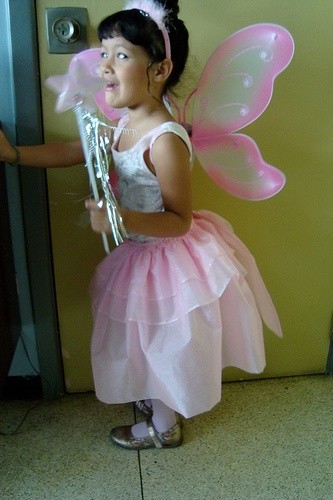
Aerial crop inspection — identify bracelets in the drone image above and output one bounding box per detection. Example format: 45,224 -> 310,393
10,144 -> 21,168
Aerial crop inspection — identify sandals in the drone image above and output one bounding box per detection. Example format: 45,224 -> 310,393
135,399 -> 182,427
110,416 -> 182,449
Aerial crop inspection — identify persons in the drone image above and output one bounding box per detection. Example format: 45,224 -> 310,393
0,1 -> 199,449
